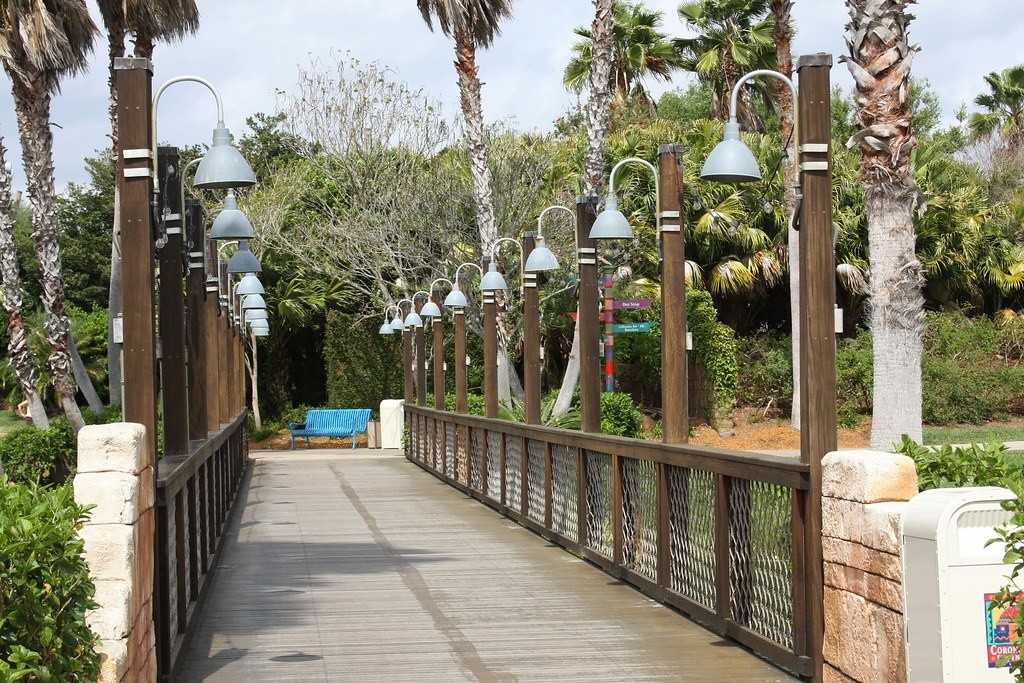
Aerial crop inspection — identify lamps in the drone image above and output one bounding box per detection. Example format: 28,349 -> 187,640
404,290 -> 434,336
524,205 -> 580,302
479,237 -> 524,314
700,69 -> 803,231
379,305 -> 404,347
420,278 -> 455,333
589,158 -> 662,273
151,75 -> 270,352
388,299 -> 412,329
444,263 -> 485,328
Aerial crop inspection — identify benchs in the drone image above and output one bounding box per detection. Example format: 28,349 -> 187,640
288,409 -> 372,450
